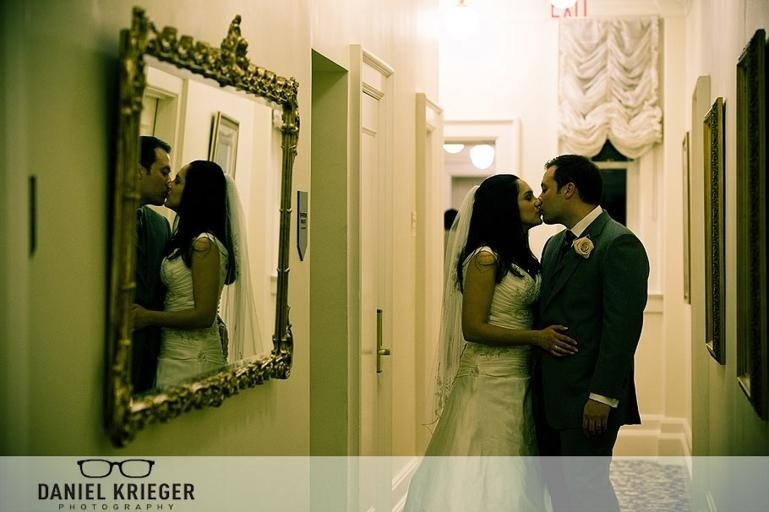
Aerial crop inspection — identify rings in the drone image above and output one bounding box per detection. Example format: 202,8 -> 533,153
553,344 -> 558,351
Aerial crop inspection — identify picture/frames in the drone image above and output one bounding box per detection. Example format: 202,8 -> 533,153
682,27 -> 769,426
209,110 -> 240,182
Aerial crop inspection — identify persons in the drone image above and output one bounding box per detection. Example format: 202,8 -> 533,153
221,12 -> 250,79
399,174 -> 580,512
130,134 -> 229,397
530,155 -> 651,512
130,160 -> 265,387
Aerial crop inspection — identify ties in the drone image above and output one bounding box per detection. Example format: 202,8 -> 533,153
136,207 -> 147,244
557,230 -> 575,262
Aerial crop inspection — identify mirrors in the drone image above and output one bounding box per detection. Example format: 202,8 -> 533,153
108,7 -> 295,440
